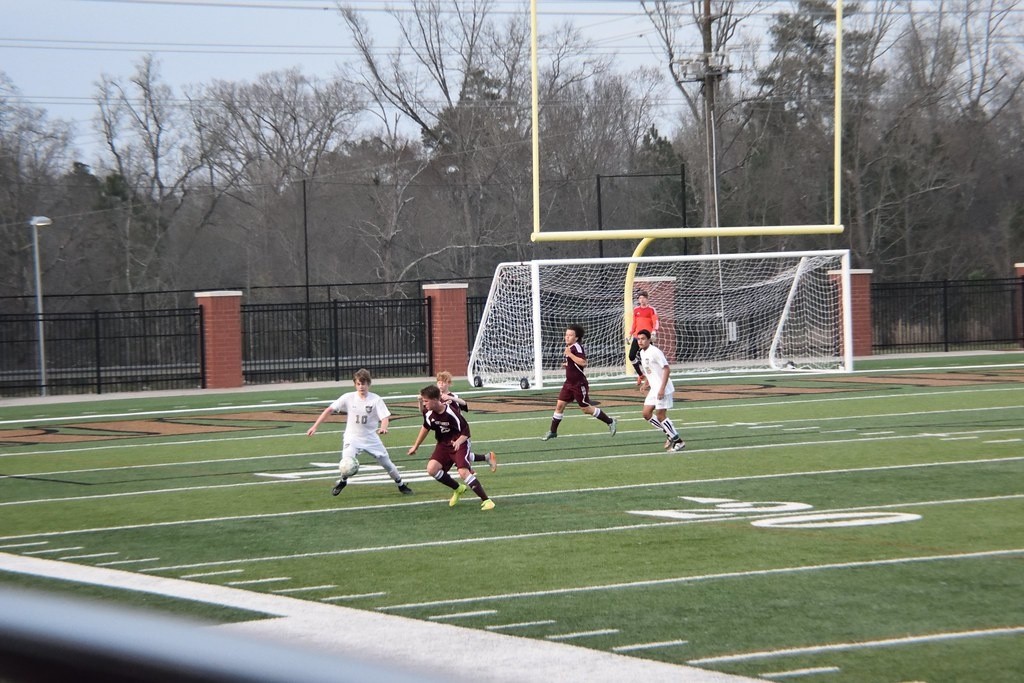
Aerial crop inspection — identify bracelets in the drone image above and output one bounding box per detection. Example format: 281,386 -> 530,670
420,401 -> 423,402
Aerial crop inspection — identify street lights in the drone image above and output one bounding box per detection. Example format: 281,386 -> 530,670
30,215 -> 53,397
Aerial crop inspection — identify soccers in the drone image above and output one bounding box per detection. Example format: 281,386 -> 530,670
337,455 -> 361,477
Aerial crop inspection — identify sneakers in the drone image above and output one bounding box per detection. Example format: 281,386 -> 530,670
332,480 -> 346,495
667,438 -> 685,452
487,452 -> 496,472
481,499 -> 496,511
541,430 -> 557,441
448,483 -> 467,507
608,418 -> 617,437
664,437 -> 671,449
637,374 -> 645,385
398,483 -> 412,495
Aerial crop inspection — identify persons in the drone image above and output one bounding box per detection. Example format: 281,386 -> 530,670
627,290 -> 659,387
538,324 -> 617,442
305,369 -> 416,496
418,371 -> 496,478
406,385 -> 495,510
637,329 -> 686,453
